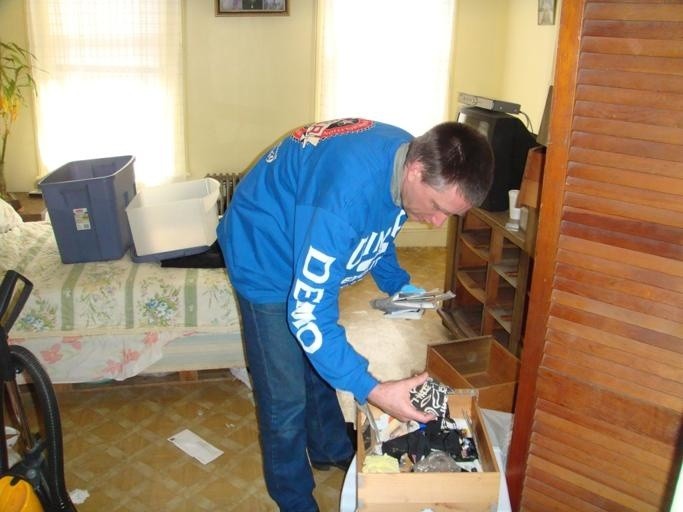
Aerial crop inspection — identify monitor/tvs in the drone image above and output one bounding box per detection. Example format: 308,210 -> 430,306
450,108 -> 542,212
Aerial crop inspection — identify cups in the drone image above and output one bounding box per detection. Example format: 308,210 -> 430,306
508,189 -> 523,221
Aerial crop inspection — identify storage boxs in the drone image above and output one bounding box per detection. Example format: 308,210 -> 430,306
356,387 -> 500,512
426,334 -> 520,413
124,178 -> 221,257
36,155 -> 136,264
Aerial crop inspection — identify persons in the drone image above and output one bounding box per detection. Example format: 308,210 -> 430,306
215,116 -> 495,512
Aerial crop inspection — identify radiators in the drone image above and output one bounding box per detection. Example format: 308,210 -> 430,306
205,172 -> 243,215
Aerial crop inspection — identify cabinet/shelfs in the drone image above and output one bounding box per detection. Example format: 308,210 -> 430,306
435,206 -> 536,362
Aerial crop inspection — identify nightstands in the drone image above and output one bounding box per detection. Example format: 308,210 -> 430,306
5,192 -> 49,221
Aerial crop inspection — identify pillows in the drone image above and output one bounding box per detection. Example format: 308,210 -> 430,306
0,196 -> 24,234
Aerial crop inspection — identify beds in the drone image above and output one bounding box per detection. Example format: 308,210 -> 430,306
0,200 -> 254,383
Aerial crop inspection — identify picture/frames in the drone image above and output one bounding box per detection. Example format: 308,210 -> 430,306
218,0 -> 287,14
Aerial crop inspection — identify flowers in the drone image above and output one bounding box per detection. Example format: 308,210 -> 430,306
0,39 -> 48,170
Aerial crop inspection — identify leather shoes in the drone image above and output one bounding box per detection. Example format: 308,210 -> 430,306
312,455 -> 353,472
